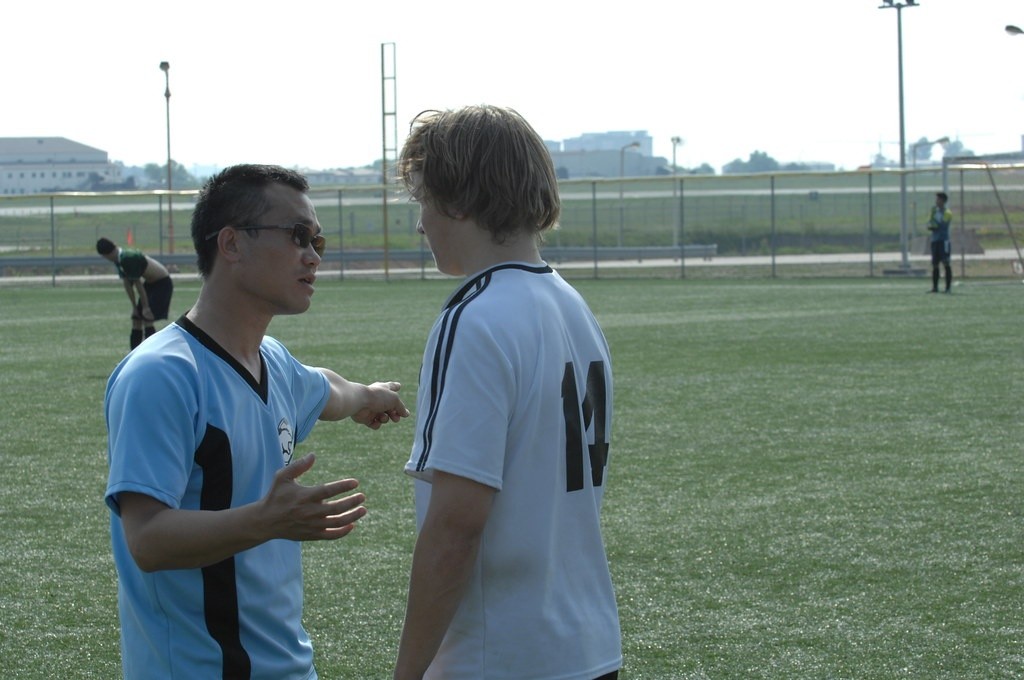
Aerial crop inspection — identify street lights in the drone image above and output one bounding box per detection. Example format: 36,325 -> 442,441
912,137 -> 949,255
671,137 -> 681,246
878,0 -> 920,269
619,142 -> 640,246
160,61 -> 174,254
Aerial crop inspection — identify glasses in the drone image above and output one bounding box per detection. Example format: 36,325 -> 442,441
204,224 -> 327,257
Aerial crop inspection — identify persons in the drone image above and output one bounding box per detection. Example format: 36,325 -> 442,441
97,238 -> 173,350
391,105 -> 623,680
926,192 -> 953,294
103,162 -> 410,680
